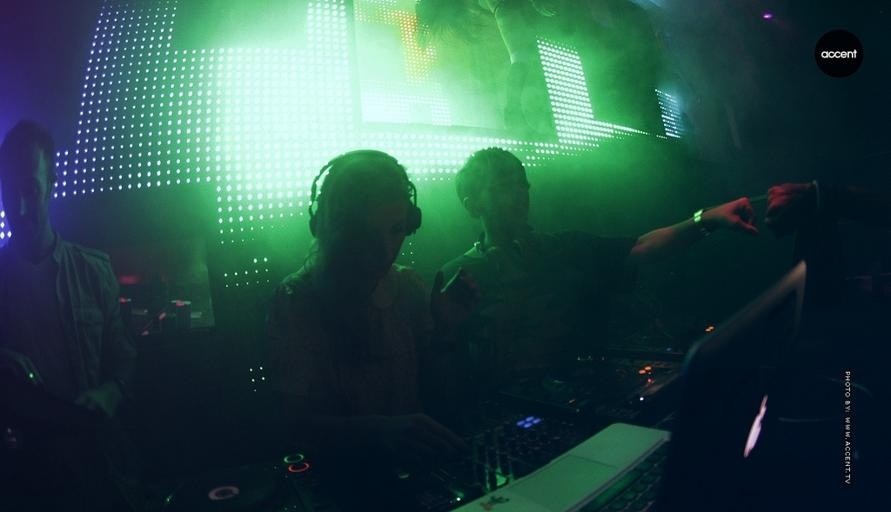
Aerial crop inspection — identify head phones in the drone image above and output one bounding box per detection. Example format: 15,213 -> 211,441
308,149 -> 422,237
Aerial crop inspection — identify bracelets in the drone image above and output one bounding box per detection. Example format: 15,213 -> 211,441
814,179 -> 822,209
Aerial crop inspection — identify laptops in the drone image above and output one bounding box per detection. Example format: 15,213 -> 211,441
658,260 -> 807,511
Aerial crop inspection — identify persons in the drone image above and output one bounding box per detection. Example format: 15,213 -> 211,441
263,151 -> 483,463
767,180 -> 888,226
429,149 -> 760,408
1,118 -> 140,510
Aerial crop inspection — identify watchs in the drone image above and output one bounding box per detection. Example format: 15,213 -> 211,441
691,204 -> 705,232
